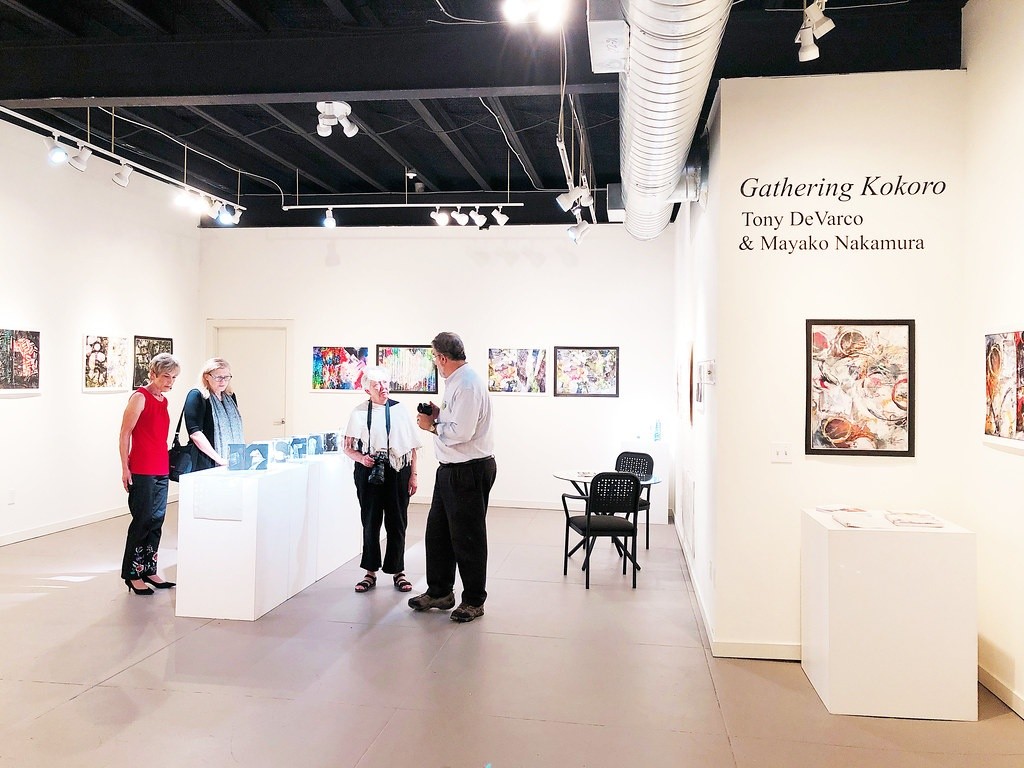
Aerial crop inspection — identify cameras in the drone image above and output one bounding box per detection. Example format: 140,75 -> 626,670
367,452 -> 385,485
418,402 -> 433,416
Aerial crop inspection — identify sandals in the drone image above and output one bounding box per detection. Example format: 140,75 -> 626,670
355,574 -> 376,591
393,573 -> 412,592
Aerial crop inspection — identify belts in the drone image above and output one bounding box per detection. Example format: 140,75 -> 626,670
439,456 -> 492,466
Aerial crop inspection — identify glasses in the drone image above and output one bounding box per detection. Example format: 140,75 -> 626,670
208,373 -> 233,382
432,353 -> 443,362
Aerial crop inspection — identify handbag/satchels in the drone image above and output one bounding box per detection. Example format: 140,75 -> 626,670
167,444 -> 193,481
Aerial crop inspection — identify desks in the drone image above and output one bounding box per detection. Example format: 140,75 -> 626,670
552,468 -> 664,572
797,502 -> 985,725
172,448 -> 390,624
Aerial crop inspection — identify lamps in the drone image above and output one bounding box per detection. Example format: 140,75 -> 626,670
110,159 -> 135,189
171,182 -> 243,230
556,179 -> 597,247
791,0 -> 836,65
321,207 -> 337,229
308,95 -> 361,142
428,205 -> 512,230
41,134 -> 94,175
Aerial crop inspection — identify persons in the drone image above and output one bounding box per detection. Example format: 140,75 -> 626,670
341,365 -> 421,592
168,357 -> 245,482
118,353 -> 176,596
407,332 -> 496,623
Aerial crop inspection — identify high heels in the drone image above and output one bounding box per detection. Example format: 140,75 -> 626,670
125,578 -> 155,595
142,575 -> 176,589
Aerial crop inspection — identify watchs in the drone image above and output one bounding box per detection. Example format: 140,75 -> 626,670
430,423 -> 439,433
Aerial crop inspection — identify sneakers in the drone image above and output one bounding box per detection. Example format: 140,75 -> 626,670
409,588 -> 455,612
450,603 -> 484,622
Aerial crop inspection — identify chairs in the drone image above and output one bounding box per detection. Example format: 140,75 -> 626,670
560,449 -> 655,593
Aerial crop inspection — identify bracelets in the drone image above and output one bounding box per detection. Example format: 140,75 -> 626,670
411,472 -> 417,475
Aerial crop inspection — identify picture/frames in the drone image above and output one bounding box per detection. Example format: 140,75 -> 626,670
75,329 -> 180,398
979,333 -> 1024,455
226,426 -> 348,474
801,314 -> 923,460
305,340 -> 441,397
0,324 -> 46,397
481,342 -> 622,400
671,336 -> 696,432
